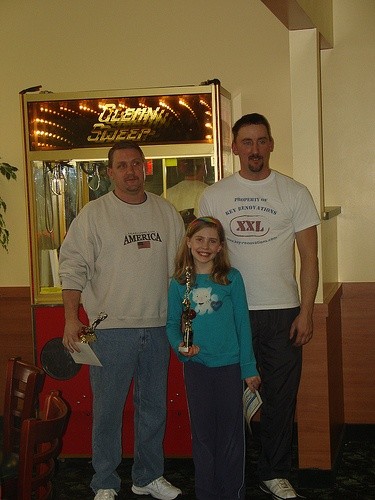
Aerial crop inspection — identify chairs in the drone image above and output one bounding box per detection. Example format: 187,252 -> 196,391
0,356 -> 70,500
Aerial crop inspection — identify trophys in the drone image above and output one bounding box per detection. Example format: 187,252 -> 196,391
77,311 -> 108,344
178,266 -> 196,353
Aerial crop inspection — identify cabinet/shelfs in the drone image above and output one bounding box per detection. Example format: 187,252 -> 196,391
18,79 -> 234,470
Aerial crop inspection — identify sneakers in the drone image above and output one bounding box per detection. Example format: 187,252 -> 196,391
93,488 -> 118,500
132,477 -> 184,500
259,478 -> 297,500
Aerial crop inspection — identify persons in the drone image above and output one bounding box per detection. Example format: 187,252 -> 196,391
198,113 -> 322,500
58,140 -> 187,500
165,216 -> 261,499
160,158 -> 211,232
